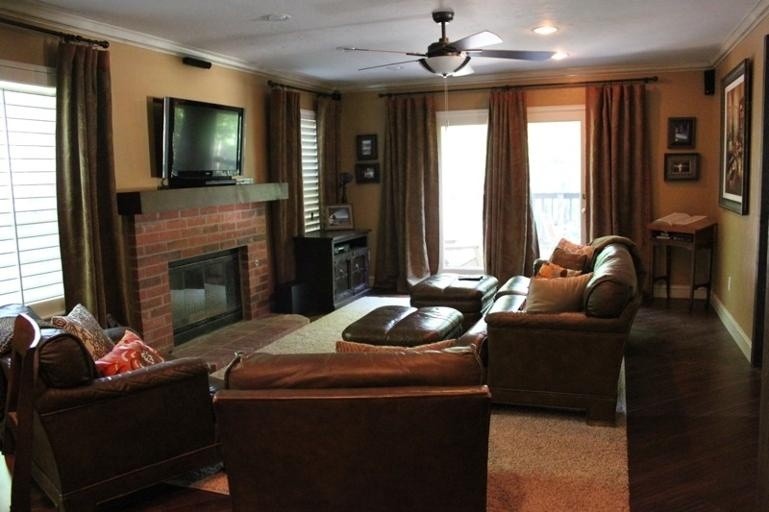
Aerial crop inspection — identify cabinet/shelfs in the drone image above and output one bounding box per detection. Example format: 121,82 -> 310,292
333,253 -> 372,302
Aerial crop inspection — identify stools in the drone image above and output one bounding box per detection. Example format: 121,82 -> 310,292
341,306 -> 465,347
410,272 -> 499,326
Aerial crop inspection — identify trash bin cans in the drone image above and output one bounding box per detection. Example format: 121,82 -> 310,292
274,279 -> 310,314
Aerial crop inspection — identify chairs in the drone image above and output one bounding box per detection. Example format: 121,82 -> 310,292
0,302 -> 224,511
210,343 -> 492,512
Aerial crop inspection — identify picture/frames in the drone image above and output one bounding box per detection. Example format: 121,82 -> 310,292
664,153 -> 699,181
325,204 -> 354,230
667,117 -> 696,148
719,58 -> 752,216
355,162 -> 380,184
356,133 -> 378,160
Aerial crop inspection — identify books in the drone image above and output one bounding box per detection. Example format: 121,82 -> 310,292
656,233 -> 692,241
654,212 -> 707,226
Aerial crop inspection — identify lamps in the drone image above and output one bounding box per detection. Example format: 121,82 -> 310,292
338,172 -> 354,205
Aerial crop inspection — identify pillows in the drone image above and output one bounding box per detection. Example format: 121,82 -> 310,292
549,248 -> 587,271
94,329 -> 165,376
51,303 -> 116,361
526,272 -> 594,312
519,259 -> 582,310
557,239 -> 595,262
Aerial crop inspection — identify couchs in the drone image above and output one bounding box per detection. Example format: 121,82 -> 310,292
484,234 -> 647,418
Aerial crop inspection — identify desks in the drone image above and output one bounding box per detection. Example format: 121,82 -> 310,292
645,216 -> 718,319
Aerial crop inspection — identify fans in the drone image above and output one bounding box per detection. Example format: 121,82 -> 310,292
337,0 -> 557,79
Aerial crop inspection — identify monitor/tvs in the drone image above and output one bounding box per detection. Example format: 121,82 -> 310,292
159,95 -> 246,185
